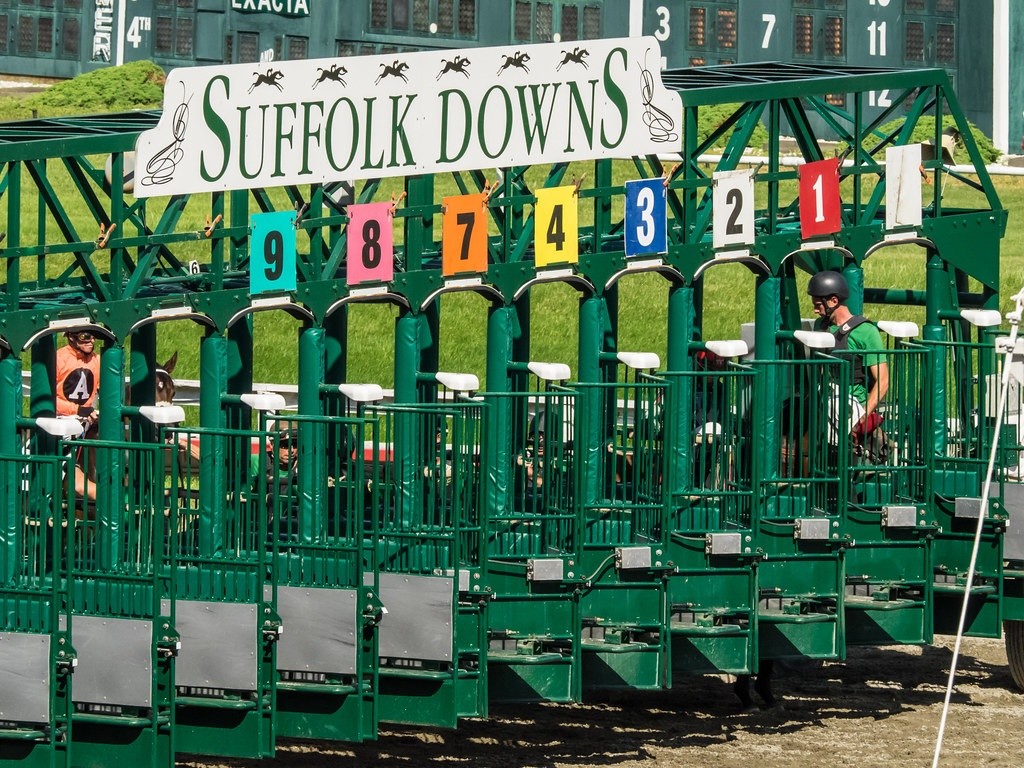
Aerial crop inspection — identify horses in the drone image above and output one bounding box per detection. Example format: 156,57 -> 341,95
704,383 -> 893,494
21,351 -> 178,536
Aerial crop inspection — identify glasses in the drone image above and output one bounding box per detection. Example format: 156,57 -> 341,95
79,334 -> 96,340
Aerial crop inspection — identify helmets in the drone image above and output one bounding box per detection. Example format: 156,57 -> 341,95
697,350 -> 732,372
807,271 -> 849,299
437,416 -> 448,434
345,425 -> 355,455
269,420 -> 298,440
529,411 -> 558,438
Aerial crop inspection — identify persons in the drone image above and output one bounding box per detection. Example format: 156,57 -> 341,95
56,329 -> 99,502
522,433 -> 546,490
236,420 -> 298,552
425,420 -> 453,488
807,271 -> 888,510
